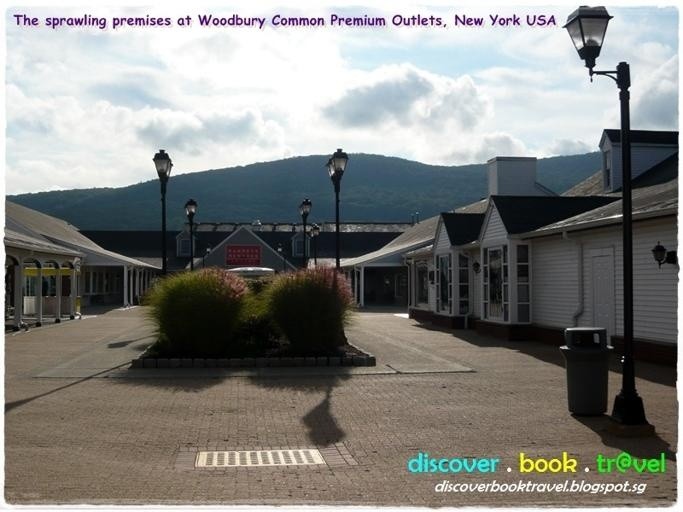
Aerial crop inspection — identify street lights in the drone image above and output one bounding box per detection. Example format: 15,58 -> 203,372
327,151 -> 347,268
185,197 -> 198,273
311,225 -> 320,266
154,148 -> 169,279
562,5 -> 644,422
298,197 -> 312,269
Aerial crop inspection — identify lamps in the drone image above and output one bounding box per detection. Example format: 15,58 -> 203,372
472,260 -> 481,275
651,240 -> 677,268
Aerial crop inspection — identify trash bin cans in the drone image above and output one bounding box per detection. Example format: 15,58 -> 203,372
559,327 -> 614,414
